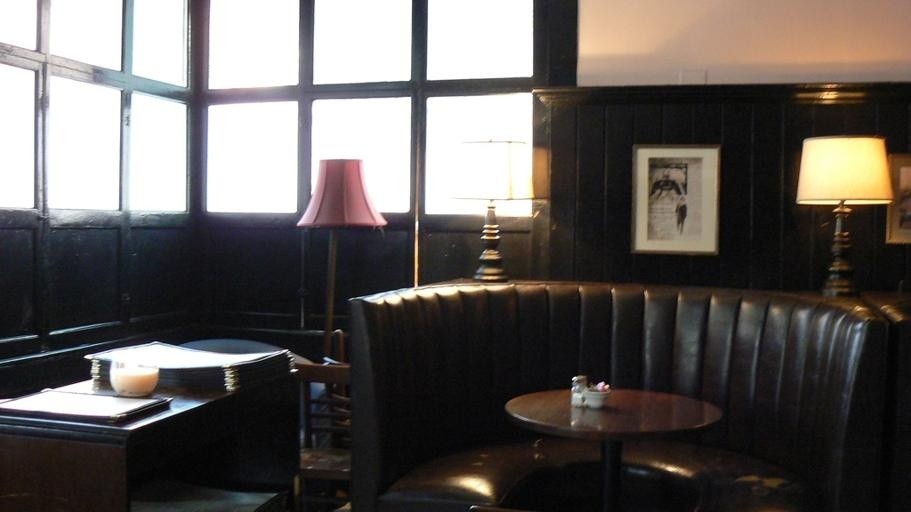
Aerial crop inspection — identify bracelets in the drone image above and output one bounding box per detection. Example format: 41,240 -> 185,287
109,362 -> 160,397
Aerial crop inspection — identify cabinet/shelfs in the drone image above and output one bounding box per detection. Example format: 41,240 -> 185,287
570,376 -> 588,407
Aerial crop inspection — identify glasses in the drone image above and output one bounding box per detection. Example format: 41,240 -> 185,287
796,136 -> 894,298
454,136 -> 534,281
296,158 -> 388,368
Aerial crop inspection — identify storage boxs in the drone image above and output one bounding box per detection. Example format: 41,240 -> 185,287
343,280 -> 890,511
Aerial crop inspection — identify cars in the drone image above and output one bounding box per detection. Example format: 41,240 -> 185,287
584,391 -> 611,409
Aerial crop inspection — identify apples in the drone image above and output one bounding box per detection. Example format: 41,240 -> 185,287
110,362 -> 160,398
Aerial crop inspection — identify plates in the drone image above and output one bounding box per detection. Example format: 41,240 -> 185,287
1,369 -> 303,512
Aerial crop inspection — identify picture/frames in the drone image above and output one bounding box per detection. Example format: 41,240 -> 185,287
503,390 -> 725,511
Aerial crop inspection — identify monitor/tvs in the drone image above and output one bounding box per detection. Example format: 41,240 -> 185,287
0,388 -> 175,427
81,341 -> 297,392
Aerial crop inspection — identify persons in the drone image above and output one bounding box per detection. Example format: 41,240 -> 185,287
675,195 -> 690,234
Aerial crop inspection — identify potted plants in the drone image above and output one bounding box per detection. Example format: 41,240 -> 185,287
883,152 -> 910,245
630,141 -> 723,256
